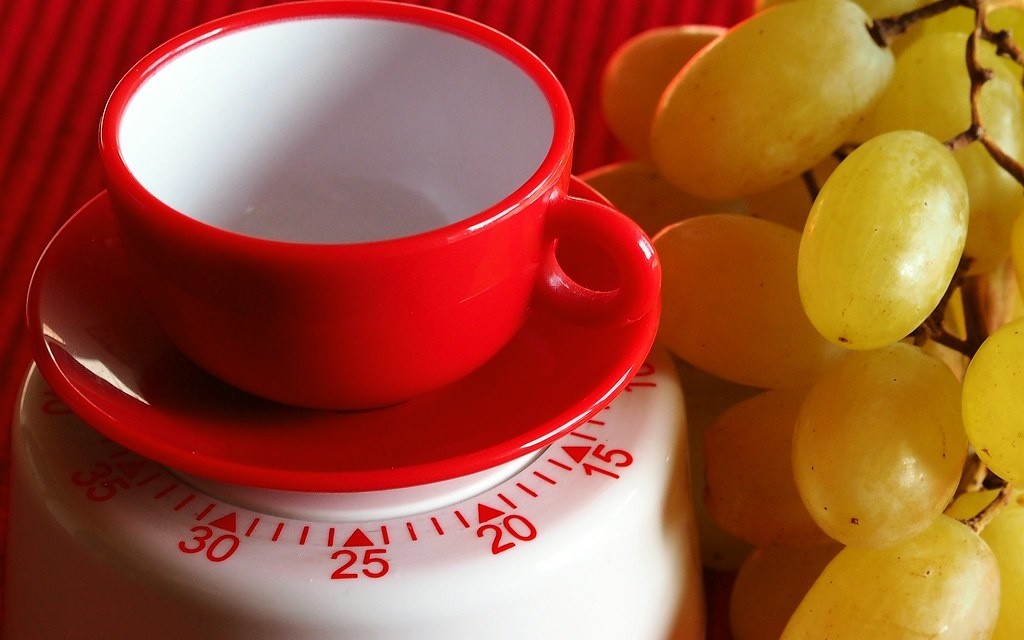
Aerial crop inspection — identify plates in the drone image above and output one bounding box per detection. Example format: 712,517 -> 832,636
30,176 -> 663,494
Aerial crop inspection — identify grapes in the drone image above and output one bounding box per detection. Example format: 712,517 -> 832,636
577,0 -> 1024,640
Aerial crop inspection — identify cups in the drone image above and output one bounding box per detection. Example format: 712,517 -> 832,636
99,0 -> 658,412
